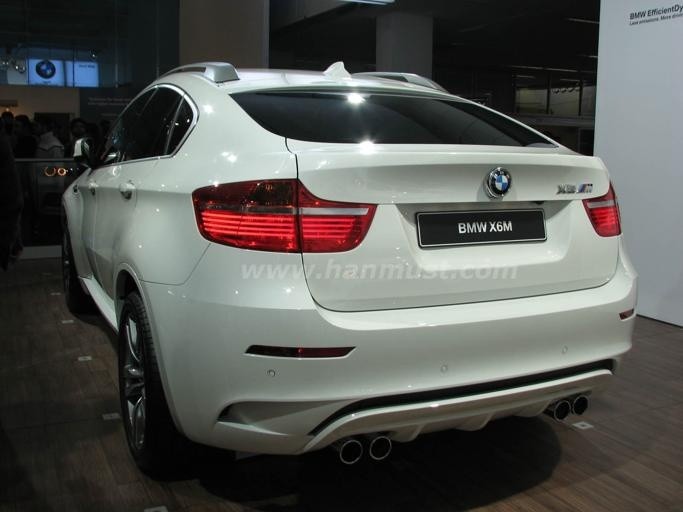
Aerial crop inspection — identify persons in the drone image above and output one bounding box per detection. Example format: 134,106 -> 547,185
0,112 -> 111,271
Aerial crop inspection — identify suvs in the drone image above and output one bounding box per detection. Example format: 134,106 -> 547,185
59,59 -> 640,480
